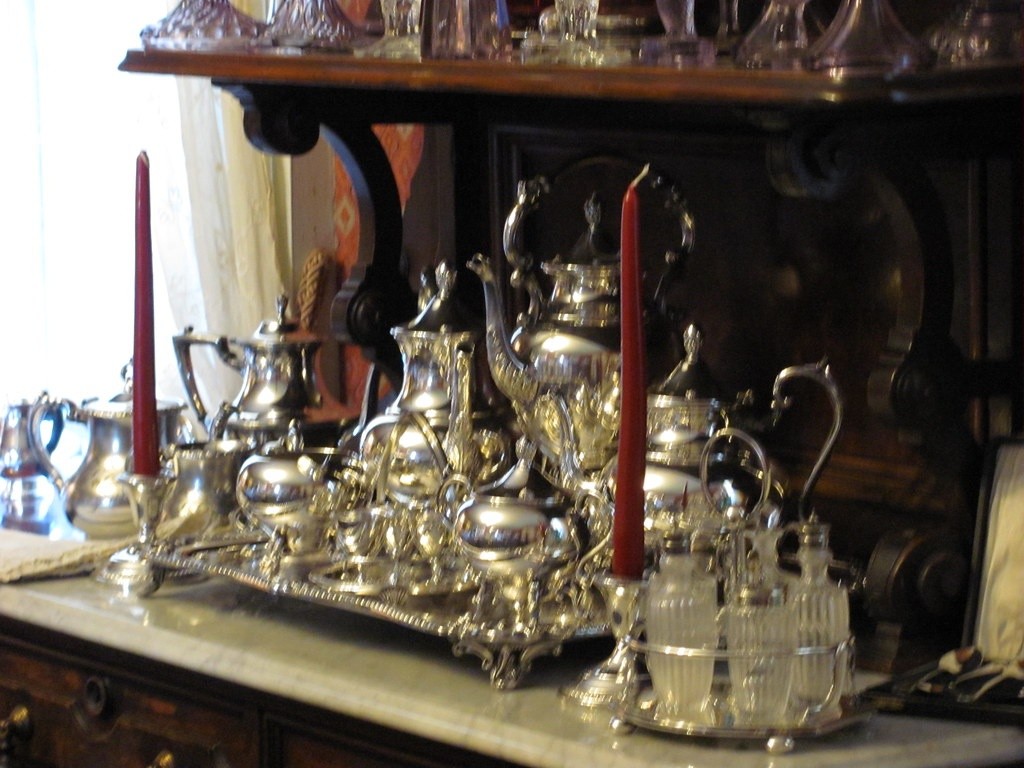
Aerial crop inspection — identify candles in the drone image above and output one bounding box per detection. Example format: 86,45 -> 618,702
131,149 -> 160,476
612,161 -> 649,580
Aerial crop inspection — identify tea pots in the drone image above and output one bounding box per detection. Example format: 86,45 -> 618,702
1,165 -> 843,602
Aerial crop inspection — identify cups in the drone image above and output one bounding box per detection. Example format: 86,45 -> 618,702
791,591 -> 849,710
641,573 -> 714,712
729,587 -> 801,719
555,0 -> 600,48
381,0 -> 422,56
